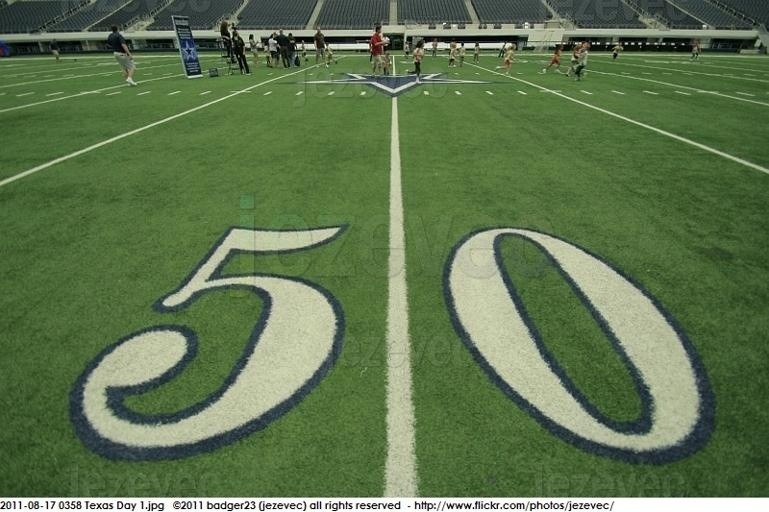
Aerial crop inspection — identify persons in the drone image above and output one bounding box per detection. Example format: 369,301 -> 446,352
219,17 -> 590,82
611,42 -> 625,60
50,38 -> 62,62
106,25 -> 138,87
689,39 -> 701,60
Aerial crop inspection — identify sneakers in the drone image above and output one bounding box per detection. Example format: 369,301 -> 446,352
126,77 -> 137,86
